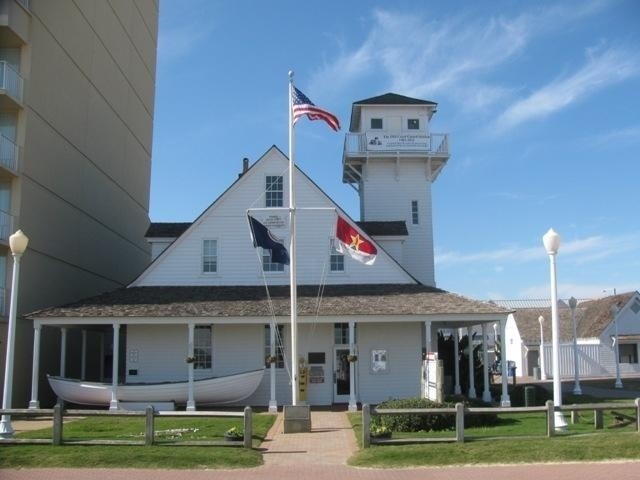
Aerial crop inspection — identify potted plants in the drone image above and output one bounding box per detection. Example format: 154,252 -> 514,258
223,426 -> 245,447
370,418 -> 394,439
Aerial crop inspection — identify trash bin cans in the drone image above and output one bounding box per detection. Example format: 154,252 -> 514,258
525,386 -> 535,407
533,367 -> 540,380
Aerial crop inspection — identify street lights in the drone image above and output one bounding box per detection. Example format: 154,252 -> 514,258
538,316 -> 547,381
543,228 -> 568,433
569,297 -> 582,396
611,305 -> 623,389
0,229 -> 29,438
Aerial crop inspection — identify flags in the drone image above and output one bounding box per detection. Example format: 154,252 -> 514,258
292,85 -> 340,132
334,215 -> 378,265
248,215 -> 290,265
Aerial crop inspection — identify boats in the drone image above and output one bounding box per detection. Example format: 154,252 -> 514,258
46,370 -> 264,407
